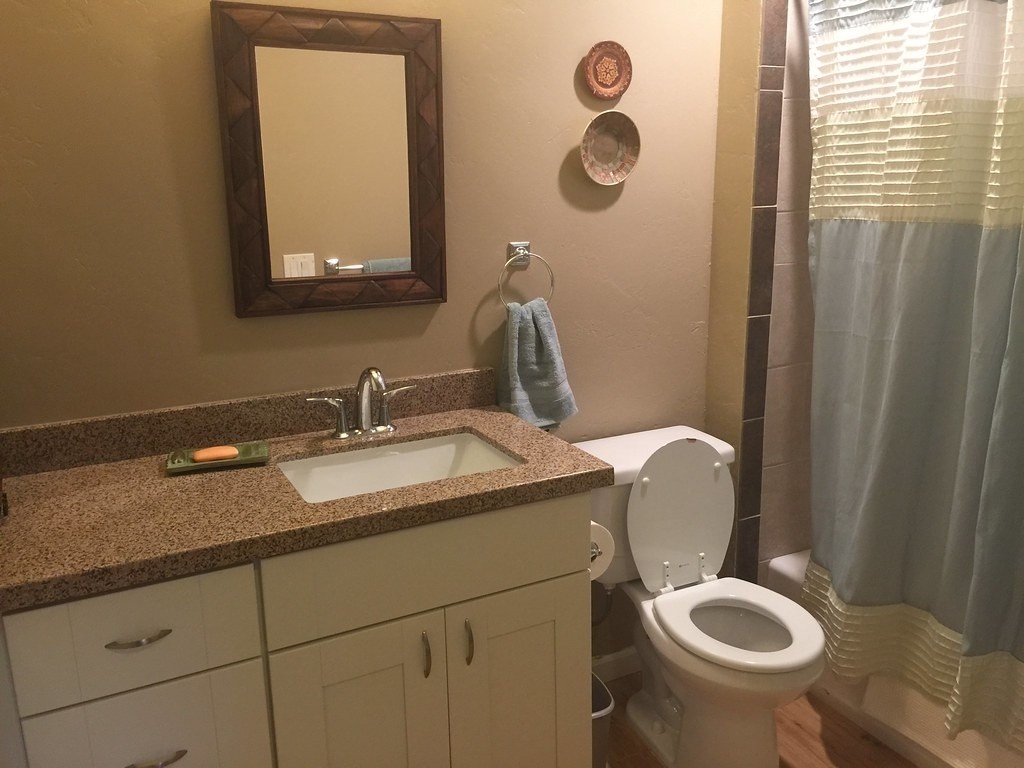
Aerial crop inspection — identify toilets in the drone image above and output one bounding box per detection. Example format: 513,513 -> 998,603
557,425 -> 833,768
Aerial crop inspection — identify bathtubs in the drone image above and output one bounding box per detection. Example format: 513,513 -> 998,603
769,521 -> 1023,768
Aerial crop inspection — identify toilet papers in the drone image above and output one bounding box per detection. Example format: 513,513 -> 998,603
589,518 -> 616,584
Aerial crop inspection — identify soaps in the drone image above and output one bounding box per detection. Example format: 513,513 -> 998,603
193,445 -> 241,463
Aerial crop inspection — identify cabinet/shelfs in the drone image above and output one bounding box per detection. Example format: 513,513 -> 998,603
2,492 -> 596,768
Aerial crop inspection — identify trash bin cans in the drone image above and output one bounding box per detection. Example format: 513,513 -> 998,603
592,670 -> 615,765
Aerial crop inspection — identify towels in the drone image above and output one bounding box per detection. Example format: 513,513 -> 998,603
503,296 -> 579,431
362,255 -> 412,276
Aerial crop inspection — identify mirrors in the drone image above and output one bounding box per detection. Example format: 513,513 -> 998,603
207,0 -> 451,321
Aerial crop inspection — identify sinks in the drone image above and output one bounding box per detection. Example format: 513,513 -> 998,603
274,425 -> 535,508
